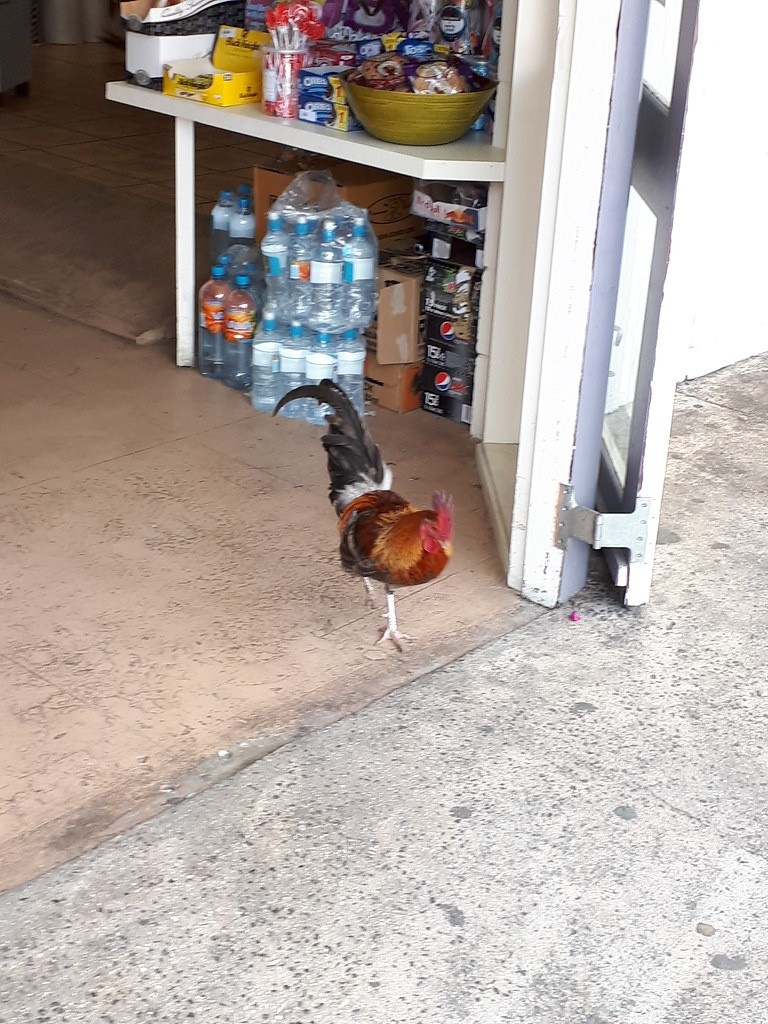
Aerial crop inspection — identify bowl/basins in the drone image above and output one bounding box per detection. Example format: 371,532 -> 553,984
338,67 -> 500,145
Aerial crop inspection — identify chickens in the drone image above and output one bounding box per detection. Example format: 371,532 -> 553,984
271,377 -> 458,656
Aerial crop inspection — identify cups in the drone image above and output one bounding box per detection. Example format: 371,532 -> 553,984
261,46 -> 307,117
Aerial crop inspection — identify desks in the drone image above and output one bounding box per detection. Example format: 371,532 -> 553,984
104,80 -> 506,368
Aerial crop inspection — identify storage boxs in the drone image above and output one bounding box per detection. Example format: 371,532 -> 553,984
298,91 -> 364,132
422,387 -> 471,426
162,24 -> 273,107
425,338 -> 475,378
365,349 -> 426,415
425,312 -> 477,351
423,220 -> 486,275
408,179 -> 489,232
253,155 -> 427,251
125,0 -> 246,92
365,231 -> 428,365
297,65 -> 356,105
424,363 -> 474,406
422,260 -> 484,322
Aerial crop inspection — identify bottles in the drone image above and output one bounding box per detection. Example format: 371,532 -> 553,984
197,183 -> 379,425
